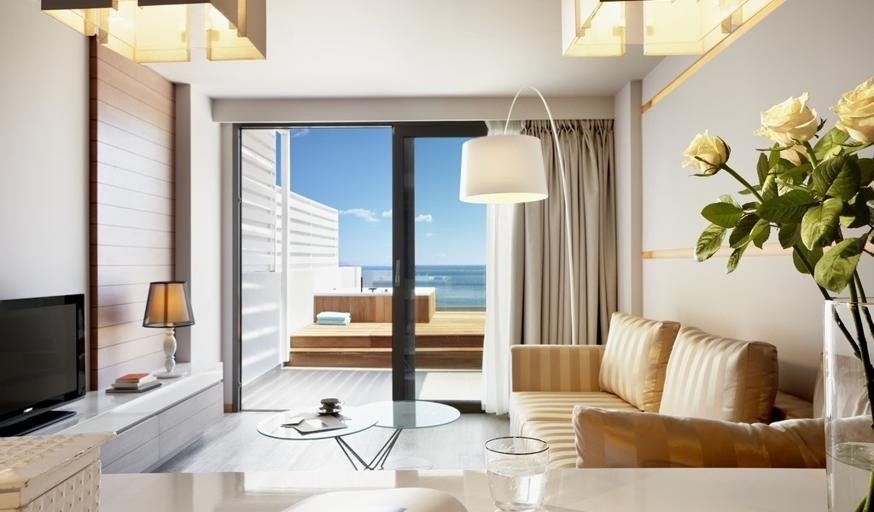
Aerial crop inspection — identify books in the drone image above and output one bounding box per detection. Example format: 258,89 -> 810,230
111,377 -> 159,389
293,414 -> 348,435
282,413 -> 303,426
105,382 -> 163,393
114,372 -> 152,383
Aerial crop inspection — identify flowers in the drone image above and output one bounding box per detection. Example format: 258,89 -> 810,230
681,75 -> 874,512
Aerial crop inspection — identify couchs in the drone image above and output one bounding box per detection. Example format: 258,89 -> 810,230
506,312 -> 874,468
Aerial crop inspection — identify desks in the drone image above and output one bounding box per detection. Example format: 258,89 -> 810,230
91,466 -> 874,512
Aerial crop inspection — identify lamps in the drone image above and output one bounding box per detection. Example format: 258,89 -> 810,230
40,0 -> 265,64
458,81 -> 578,345
560,0 -> 785,60
142,281 -> 196,379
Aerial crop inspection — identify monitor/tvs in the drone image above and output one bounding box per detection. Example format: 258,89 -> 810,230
0,294 -> 85,437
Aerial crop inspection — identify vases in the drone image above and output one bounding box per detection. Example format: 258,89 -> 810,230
823,299 -> 874,512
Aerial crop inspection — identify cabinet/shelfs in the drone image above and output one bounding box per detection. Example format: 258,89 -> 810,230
17,370 -> 224,475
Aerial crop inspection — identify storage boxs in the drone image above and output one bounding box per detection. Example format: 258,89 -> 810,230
1,431 -> 120,511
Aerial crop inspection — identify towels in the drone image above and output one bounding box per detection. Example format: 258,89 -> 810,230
316,310 -> 352,327
